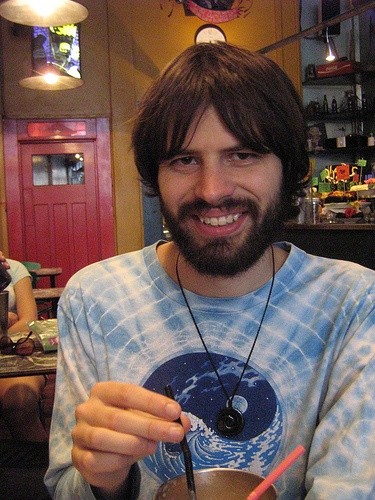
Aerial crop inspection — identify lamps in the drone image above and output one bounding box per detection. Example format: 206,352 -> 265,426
20,66 -> 84,91
326,43 -> 336,61
0,0 -> 88,27
33,25 -> 81,79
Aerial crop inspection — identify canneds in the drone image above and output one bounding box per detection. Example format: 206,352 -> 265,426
304,200 -> 318,225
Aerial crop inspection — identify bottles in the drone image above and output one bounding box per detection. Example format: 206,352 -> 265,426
347,94 -> 354,111
362,90 -> 368,110
323,95 -> 328,113
314,97 -> 320,115
368,133 -> 375,146
332,95 -> 337,113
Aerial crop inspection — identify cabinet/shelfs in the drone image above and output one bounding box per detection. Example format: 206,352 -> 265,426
301,69 -> 365,152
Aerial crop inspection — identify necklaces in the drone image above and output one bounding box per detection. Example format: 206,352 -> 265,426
176,245 -> 275,437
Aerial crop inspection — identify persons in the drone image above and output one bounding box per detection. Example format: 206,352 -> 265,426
43,42 -> 375,500
0,258 -> 48,443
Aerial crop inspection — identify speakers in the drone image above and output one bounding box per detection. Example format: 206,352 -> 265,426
318,0 -> 341,37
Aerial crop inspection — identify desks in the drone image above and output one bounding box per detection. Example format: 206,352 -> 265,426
0,268 -> 66,433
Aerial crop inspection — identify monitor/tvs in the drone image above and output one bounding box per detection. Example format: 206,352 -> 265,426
30,21 -> 81,78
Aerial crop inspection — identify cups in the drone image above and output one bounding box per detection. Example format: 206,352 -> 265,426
154,467 -> 280,500
0,262 -> 12,293
0,290 -> 9,339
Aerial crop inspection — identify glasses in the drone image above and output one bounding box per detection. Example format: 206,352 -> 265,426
0,331 -> 36,356
